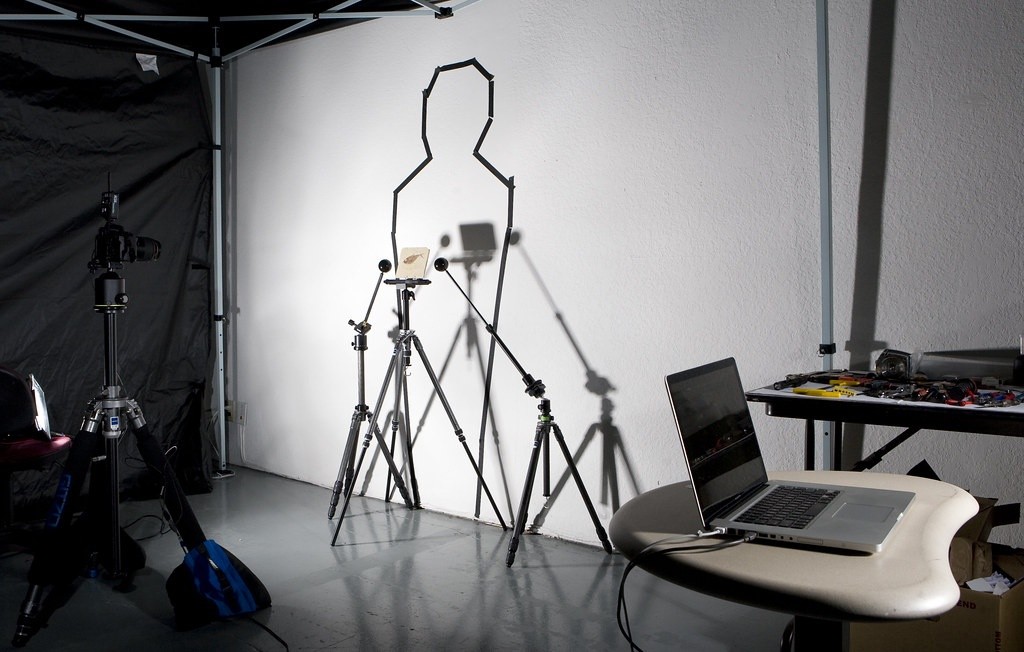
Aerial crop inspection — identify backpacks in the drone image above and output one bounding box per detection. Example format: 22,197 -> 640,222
166,540 -> 271,630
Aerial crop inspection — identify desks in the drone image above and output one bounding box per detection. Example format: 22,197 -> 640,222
609,470 -> 979,652
745,369 -> 1024,482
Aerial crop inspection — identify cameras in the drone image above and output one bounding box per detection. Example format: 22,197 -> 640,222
94,222 -> 161,261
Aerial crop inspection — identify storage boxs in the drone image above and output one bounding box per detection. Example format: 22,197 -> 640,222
849,543 -> 1024,652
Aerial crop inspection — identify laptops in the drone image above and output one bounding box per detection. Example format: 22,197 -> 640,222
663,357 -> 916,554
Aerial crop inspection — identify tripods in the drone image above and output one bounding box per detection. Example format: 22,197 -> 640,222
327,258 -> 613,568
11,259 -> 205,652
